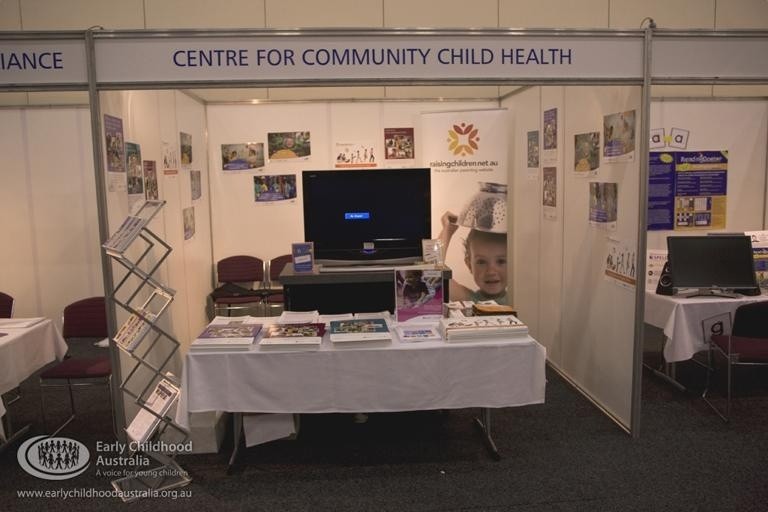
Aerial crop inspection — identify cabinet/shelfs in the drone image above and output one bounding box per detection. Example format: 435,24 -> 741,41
101,198 -> 194,504
276,260 -> 453,312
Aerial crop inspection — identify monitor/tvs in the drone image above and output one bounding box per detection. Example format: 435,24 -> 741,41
667,235 -> 757,297
302,169 -> 432,263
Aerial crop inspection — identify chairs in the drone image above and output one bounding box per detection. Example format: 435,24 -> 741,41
0,291 -> 21,405
260,253 -> 293,317
37,294 -> 118,439
212,254 -> 268,317
693,299 -> 767,423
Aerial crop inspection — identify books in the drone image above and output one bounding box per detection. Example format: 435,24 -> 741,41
112,307 -> 157,353
188,299 -> 530,352
126,379 -> 180,447
101,215 -> 146,255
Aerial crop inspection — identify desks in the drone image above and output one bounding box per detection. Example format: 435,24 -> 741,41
640,281 -> 768,397
178,313 -> 548,474
0,314 -> 72,455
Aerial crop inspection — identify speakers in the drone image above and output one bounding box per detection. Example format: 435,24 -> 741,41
655,262 -> 673,295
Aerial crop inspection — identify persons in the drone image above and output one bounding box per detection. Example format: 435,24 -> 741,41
105,127 -> 201,242
224,137 -> 304,199
403,270 -> 427,305
752,235 -> 759,242
606,247 -> 637,276
437,211 -> 508,304
386,133 -> 414,159
337,147 -> 375,163
527,109 -> 631,226
